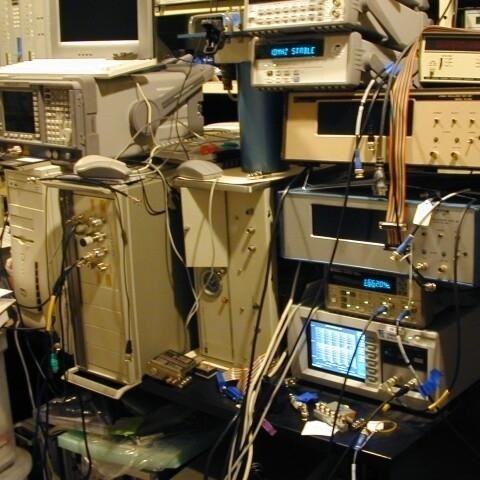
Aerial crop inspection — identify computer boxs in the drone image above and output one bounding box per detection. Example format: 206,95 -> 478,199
4,157 -> 63,332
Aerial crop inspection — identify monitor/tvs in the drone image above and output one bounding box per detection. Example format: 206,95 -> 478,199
50,0 -> 153,59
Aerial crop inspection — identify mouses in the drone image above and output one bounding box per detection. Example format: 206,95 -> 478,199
176,159 -> 222,181
73,155 -> 131,179
